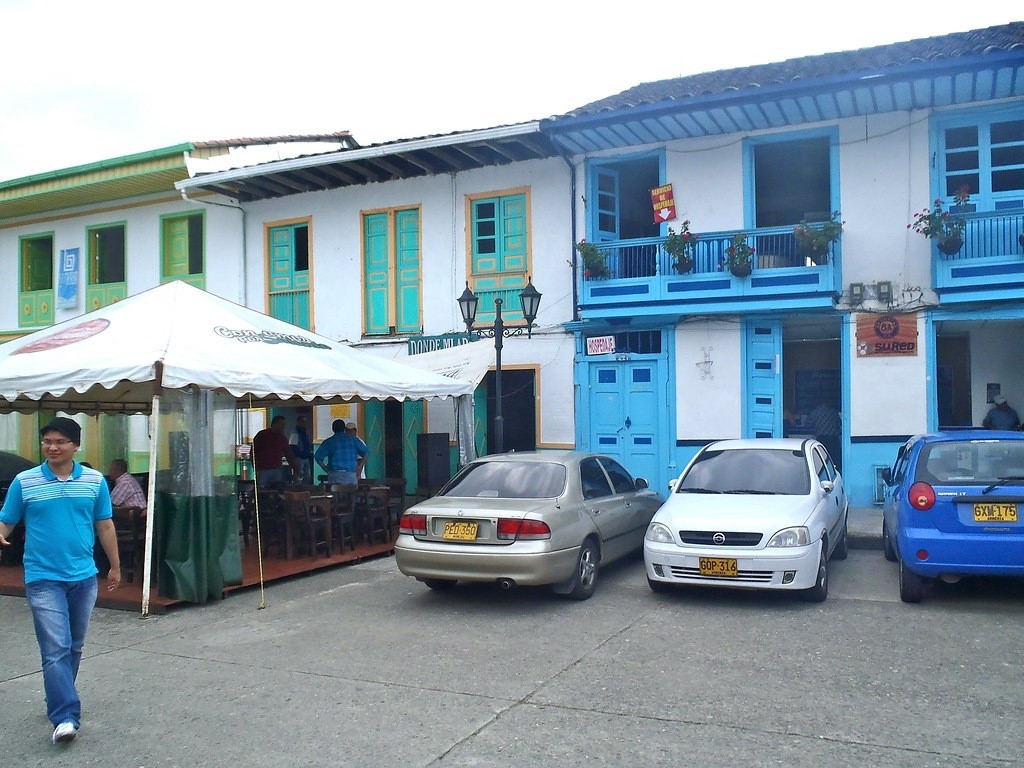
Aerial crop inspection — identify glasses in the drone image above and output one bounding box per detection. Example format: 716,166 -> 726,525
40,439 -> 75,448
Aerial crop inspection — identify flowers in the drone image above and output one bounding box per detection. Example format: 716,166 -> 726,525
567,241 -> 611,279
716,231 -> 755,274
907,184 -> 969,243
662,220 -> 699,268
792,210 -> 845,255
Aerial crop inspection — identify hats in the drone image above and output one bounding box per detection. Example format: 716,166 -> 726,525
39,417 -> 81,446
344,422 -> 357,432
994,395 -> 1008,405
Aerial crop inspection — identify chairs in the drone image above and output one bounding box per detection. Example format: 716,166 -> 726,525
104,475 -> 408,587
928,458 -> 945,471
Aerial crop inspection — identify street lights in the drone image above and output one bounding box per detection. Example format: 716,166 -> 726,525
456,276 -> 542,454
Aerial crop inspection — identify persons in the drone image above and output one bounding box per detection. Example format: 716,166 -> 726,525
983,395 -> 1021,430
107,459 -> 146,510
344,422 -> 367,480
783,395 -> 797,437
0,417 -> 121,742
314,419 -> 369,491
803,397 -> 841,466
289,416 -> 314,484
250,415 -> 301,514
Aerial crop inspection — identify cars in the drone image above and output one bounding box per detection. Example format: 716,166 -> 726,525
643,437 -> 849,604
394,450 -> 666,601
882,428 -> 1024,602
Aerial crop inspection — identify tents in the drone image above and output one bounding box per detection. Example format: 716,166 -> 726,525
0,279 -> 475,613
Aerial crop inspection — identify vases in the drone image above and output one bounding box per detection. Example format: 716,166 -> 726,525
732,264 -> 748,277
588,268 -> 604,277
677,263 -> 692,272
937,240 -> 963,254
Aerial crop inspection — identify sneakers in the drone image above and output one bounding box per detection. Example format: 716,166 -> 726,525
53,721 -> 76,743
